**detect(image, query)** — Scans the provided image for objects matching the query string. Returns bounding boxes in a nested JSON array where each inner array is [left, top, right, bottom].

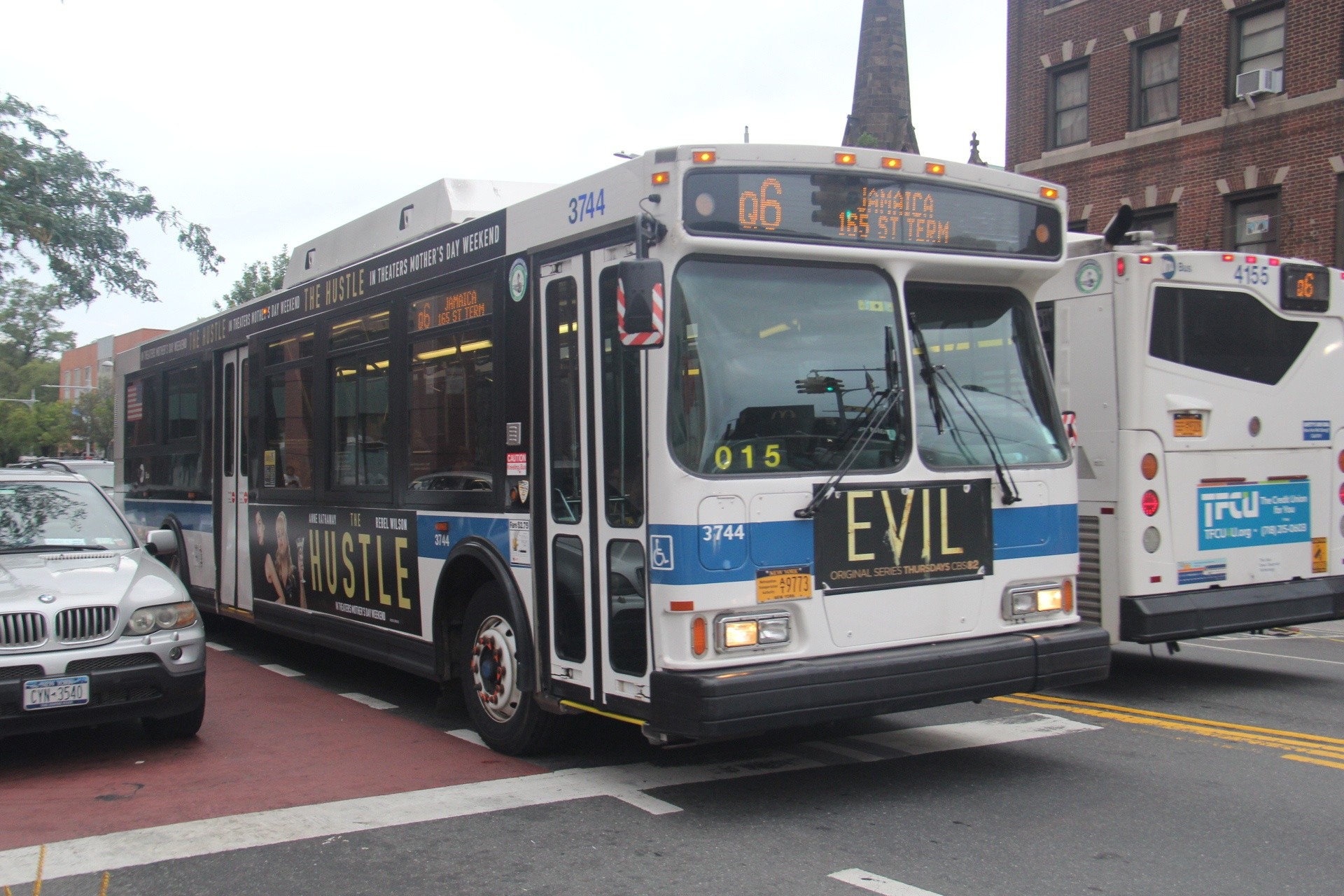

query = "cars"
[[28, 460, 116, 508]]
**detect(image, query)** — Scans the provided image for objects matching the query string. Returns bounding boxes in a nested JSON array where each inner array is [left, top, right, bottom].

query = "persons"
[[250, 509, 308, 609], [283, 466, 303, 488]]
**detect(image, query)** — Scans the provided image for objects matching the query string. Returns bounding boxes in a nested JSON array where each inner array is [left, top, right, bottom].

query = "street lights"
[[41, 366, 99, 460]]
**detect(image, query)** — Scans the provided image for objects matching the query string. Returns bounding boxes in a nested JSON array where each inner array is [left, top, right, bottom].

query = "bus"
[[107, 140, 1114, 763], [912, 203, 1343, 647]]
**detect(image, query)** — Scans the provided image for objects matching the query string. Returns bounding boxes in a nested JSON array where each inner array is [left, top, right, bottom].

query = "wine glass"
[[295, 537, 306, 583]]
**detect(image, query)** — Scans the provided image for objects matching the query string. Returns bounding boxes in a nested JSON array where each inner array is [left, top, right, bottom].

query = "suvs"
[[0, 459, 209, 754]]
